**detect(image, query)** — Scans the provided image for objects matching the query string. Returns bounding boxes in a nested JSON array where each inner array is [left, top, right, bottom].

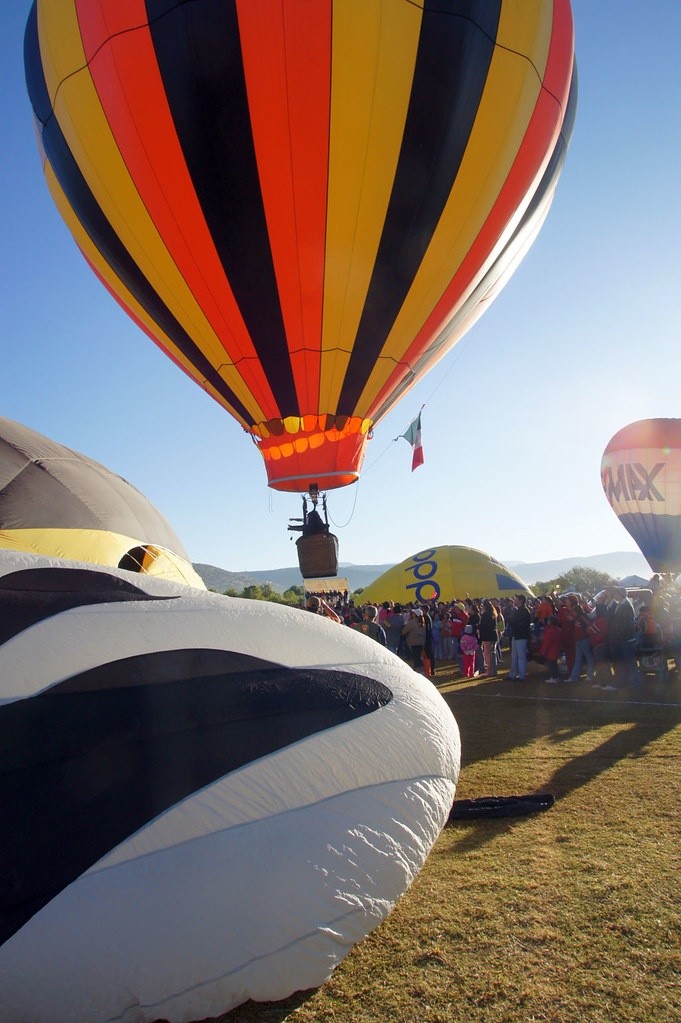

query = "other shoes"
[[563, 678, 575, 682], [602, 686, 618, 691], [592, 684, 602, 688], [584, 677, 592, 681], [503, 676, 515, 680], [513, 678, 523, 682]]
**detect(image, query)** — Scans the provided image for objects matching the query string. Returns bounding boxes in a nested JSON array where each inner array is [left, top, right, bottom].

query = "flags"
[[395, 410, 424, 472]]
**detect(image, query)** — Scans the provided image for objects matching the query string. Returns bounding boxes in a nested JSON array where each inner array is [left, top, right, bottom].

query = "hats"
[[455, 603, 465, 611], [412, 609, 423, 616], [465, 625, 473, 633], [308, 596, 321, 607]]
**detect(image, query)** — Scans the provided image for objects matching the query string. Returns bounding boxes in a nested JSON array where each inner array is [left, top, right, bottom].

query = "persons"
[[305, 575, 681, 691]]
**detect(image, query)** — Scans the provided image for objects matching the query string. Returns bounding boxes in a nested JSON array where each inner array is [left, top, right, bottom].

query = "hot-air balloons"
[[352, 546, 537, 608], [21, 0, 582, 581], [0, 414, 463, 1023], [600, 416, 681, 597]]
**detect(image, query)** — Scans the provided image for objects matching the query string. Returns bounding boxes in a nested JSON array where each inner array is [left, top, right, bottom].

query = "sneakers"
[[545, 677, 560, 683]]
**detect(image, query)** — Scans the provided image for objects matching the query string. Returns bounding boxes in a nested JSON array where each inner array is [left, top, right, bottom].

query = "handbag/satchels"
[[420, 651, 432, 676]]
